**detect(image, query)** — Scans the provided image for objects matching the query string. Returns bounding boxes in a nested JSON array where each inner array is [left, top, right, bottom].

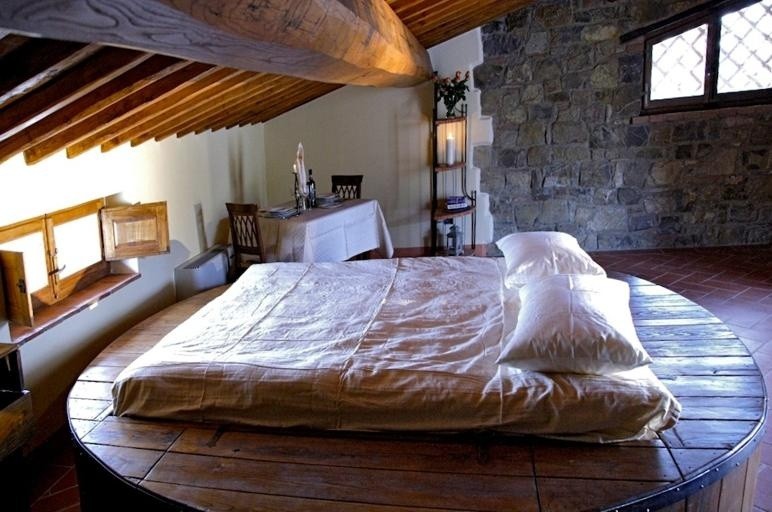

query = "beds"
[[66, 257, 768, 511]]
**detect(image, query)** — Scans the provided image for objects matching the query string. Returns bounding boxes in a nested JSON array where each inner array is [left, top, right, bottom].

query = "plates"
[[316, 193, 337, 199], [320, 203, 343, 208], [267, 207, 292, 213]]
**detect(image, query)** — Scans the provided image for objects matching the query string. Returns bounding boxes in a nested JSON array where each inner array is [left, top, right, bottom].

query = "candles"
[[446, 134, 455, 166]]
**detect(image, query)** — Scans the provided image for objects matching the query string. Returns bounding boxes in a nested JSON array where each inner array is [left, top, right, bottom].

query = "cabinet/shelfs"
[[432, 70, 476, 257]]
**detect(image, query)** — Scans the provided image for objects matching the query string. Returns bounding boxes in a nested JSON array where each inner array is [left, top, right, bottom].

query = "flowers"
[[433, 71, 470, 118]]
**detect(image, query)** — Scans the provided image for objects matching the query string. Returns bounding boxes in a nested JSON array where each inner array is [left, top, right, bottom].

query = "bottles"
[[298, 195, 303, 215], [305, 169, 316, 208]]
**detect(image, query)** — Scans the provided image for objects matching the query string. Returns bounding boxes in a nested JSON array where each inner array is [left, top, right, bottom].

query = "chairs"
[[332, 175, 362, 200], [225, 202, 266, 269]]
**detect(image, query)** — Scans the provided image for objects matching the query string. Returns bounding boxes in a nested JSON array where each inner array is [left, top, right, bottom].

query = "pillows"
[[495, 274, 653, 376], [495, 232, 606, 290]]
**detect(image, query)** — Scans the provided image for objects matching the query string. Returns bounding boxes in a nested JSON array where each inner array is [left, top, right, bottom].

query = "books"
[[258, 206, 298, 219], [317, 192, 345, 209]]
